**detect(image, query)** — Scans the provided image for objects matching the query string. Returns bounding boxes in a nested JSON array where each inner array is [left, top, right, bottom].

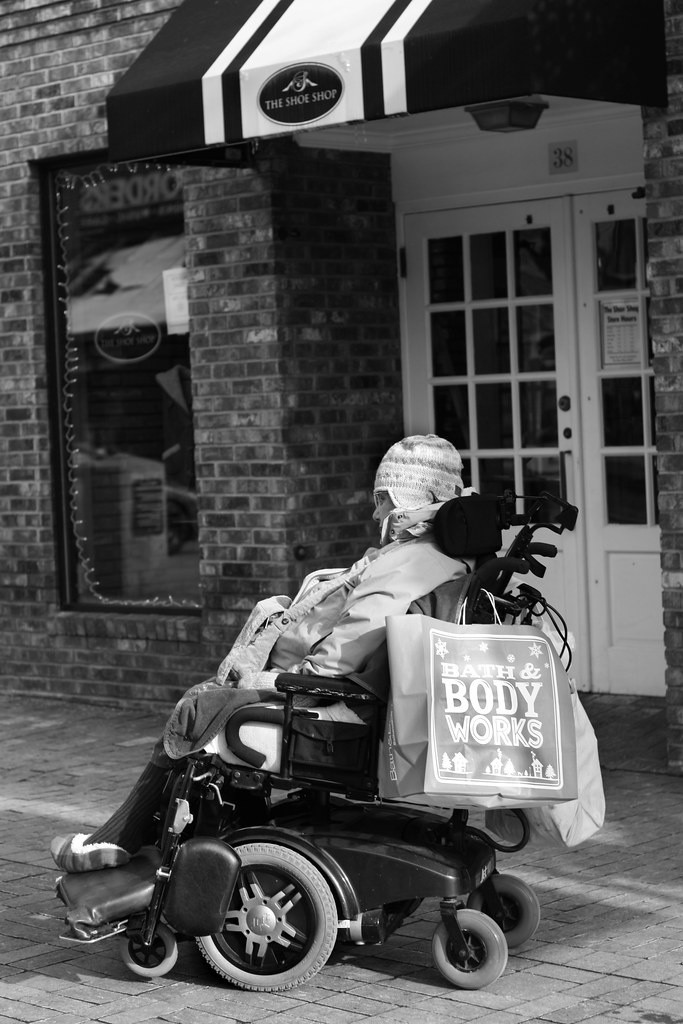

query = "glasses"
[[374, 490, 388, 508]]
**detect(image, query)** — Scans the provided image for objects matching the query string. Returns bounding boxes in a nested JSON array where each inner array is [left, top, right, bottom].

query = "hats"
[[372, 434, 463, 512]]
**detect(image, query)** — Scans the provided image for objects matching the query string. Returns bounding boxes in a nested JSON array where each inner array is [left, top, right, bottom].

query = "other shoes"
[[51, 831, 131, 871]]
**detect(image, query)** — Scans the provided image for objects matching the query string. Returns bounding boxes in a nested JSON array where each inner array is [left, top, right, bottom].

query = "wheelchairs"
[[52, 488, 580, 997]]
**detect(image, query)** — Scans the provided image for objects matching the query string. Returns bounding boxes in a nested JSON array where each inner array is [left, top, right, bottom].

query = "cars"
[[73, 438, 197, 557]]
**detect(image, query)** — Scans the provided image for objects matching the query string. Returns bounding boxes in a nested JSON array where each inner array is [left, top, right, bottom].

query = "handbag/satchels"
[[216, 595, 295, 684], [378, 587, 605, 846]]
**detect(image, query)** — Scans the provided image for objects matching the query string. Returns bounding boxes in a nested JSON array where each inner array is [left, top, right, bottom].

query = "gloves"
[[235, 663, 318, 707]]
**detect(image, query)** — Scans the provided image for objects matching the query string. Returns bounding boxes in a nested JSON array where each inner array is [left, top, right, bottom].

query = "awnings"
[[106, 0, 667, 166]]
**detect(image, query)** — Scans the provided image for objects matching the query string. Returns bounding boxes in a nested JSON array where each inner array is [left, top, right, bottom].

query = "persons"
[[48, 434, 474, 871]]
